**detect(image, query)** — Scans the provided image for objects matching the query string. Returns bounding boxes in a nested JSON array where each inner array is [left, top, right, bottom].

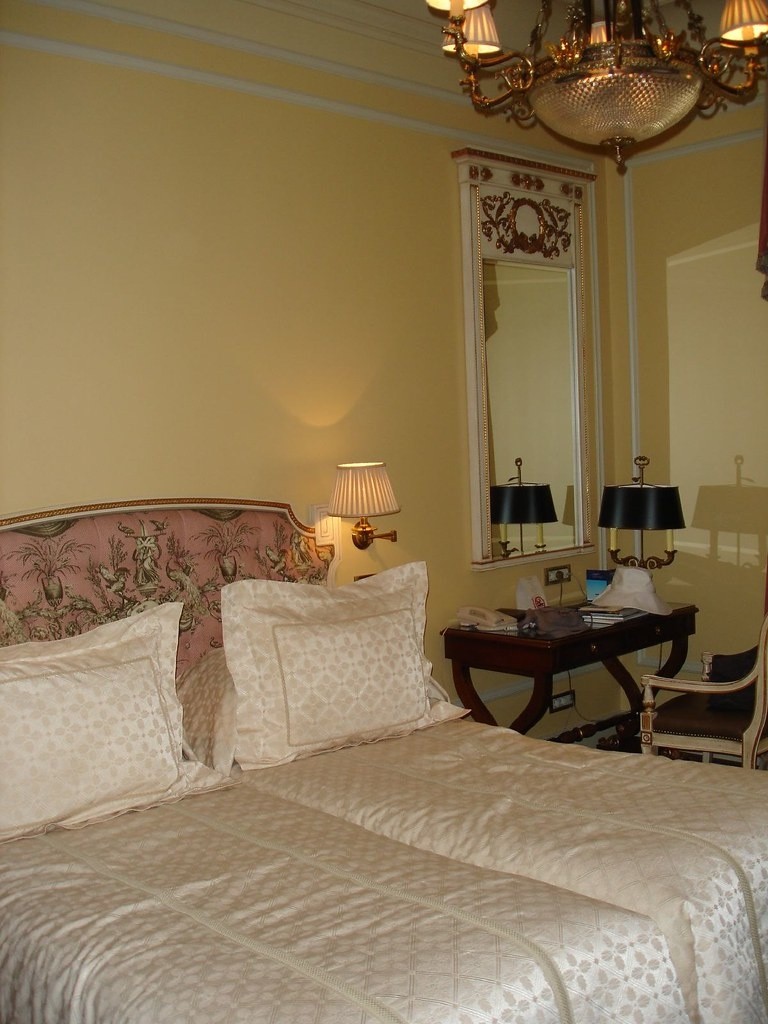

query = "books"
[[580, 609, 648, 620], [584, 619, 621, 625]]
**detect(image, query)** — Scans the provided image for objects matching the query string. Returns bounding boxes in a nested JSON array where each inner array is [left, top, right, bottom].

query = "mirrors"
[[450, 146, 597, 572]]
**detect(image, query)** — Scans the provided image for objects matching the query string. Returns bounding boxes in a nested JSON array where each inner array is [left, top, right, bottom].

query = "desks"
[[439, 597, 699, 759]]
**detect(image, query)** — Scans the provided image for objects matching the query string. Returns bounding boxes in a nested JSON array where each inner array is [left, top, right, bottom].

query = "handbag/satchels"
[[517, 606, 589, 640]]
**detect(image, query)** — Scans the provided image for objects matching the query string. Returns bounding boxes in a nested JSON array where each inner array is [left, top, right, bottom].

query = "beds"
[[0, 497, 768, 1024]]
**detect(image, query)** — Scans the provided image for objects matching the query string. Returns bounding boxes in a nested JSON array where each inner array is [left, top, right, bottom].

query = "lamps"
[[490, 456, 558, 558], [597, 456, 686, 570], [328, 461, 401, 551], [425, 0, 768, 166]]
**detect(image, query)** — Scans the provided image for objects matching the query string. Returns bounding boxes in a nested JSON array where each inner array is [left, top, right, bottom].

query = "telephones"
[[455, 605, 517, 628]]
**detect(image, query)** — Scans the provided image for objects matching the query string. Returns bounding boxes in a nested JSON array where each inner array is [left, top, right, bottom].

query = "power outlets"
[[543, 564, 571, 587], [548, 689, 576, 714]]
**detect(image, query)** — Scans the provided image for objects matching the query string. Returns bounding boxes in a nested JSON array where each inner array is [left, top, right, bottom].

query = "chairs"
[[634, 611, 768, 769]]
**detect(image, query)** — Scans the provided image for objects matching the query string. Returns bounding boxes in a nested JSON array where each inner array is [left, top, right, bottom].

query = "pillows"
[[0, 601, 241, 844], [220, 560, 472, 770]]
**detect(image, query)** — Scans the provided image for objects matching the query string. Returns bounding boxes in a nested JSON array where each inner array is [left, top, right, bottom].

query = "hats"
[[591, 564, 673, 616]]
